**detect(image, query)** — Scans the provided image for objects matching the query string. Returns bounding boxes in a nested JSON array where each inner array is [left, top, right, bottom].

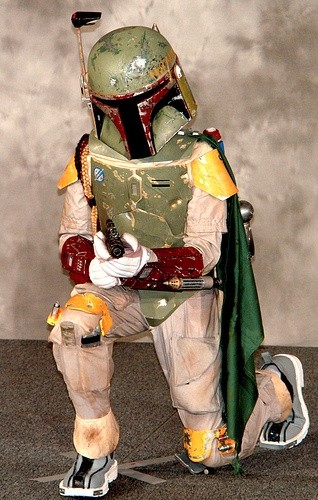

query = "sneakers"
[[58, 449, 118, 498], [259, 352, 309, 450]]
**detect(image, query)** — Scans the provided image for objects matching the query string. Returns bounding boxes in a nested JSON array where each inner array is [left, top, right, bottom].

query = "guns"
[[104, 218, 125, 259]]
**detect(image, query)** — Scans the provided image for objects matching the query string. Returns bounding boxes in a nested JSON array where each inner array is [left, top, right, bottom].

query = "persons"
[[45, 11, 311, 499]]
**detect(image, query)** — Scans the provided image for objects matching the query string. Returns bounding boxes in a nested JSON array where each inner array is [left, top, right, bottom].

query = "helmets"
[[84, 25, 196, 160]]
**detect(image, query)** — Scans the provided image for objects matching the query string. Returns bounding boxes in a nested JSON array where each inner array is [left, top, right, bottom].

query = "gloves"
[[89, 257, 121, 289], [93, 230, 158, 277]]
